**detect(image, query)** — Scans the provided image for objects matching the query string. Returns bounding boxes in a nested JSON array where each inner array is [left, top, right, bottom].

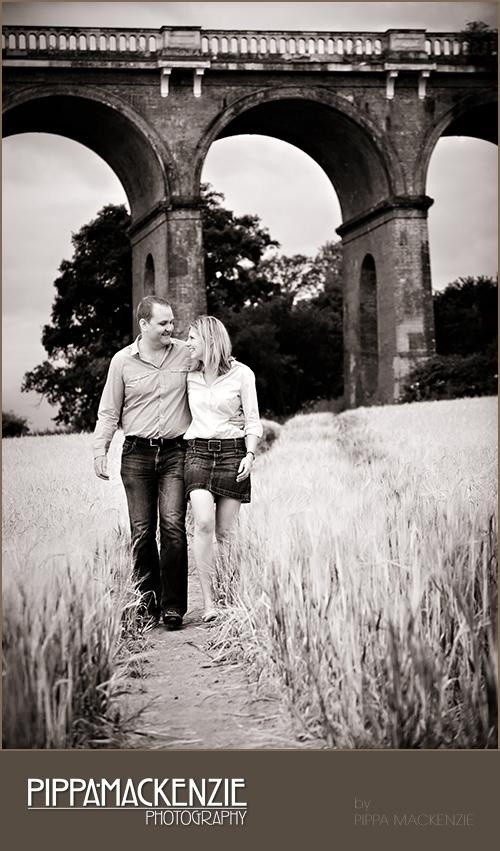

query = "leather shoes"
[[163, 609, 184, 626]]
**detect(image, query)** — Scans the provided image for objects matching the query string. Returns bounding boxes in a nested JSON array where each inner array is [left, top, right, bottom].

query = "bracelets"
[[247, 452, 256, 460]]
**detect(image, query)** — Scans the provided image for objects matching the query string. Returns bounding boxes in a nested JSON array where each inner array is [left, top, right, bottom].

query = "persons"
[[178, 315, 266, 622], [91, 291, 246, 628]]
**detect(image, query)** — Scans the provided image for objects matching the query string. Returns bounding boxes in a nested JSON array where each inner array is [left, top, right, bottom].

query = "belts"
[[186, 439, 243, 451], [125, 436, 182, 447]]
